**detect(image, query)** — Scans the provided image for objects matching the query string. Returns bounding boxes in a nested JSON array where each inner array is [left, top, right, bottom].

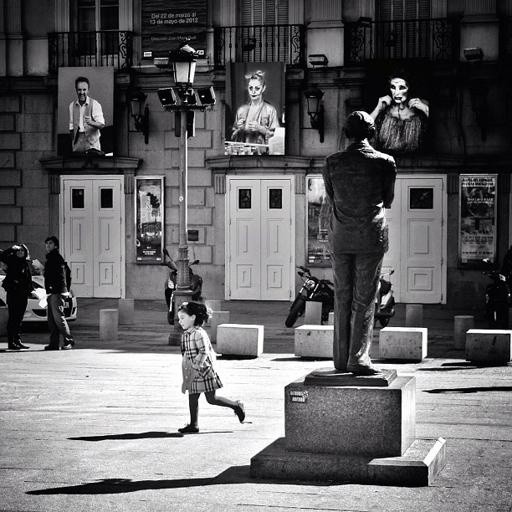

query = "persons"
[[320, 111, 397, 376], [232, 70, 279, 154], [369, 75, 430, 154], [42, 234, 74, 350], [1, 243, 32, 350], [176, 301, 245, 433], [67, 76, 106, 151]]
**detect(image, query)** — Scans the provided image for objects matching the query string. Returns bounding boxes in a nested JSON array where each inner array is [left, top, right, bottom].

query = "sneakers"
[[61, 341, 75, 350], [177, 424, 200, 434], [44, 344, 60, 351], [8, 338, 31, 350], [234, 400, 246, 424]]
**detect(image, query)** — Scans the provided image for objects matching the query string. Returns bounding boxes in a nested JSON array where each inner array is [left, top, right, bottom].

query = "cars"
[[137, 222, 161, 257], [0, 248, 79, 324]]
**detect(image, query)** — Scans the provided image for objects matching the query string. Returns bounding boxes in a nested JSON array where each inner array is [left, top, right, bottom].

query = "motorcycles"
[[159, 250, 202, 324], [283, 265, 396, 327], [481, 257, 512, 328]]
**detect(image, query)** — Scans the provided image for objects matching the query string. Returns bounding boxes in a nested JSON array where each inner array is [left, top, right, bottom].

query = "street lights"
[[159, 38, 217, 345]]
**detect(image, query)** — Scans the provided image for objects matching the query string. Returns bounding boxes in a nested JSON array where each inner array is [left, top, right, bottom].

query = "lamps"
[[126, 90, 152, 145], [304, 82, 327, 144]]
